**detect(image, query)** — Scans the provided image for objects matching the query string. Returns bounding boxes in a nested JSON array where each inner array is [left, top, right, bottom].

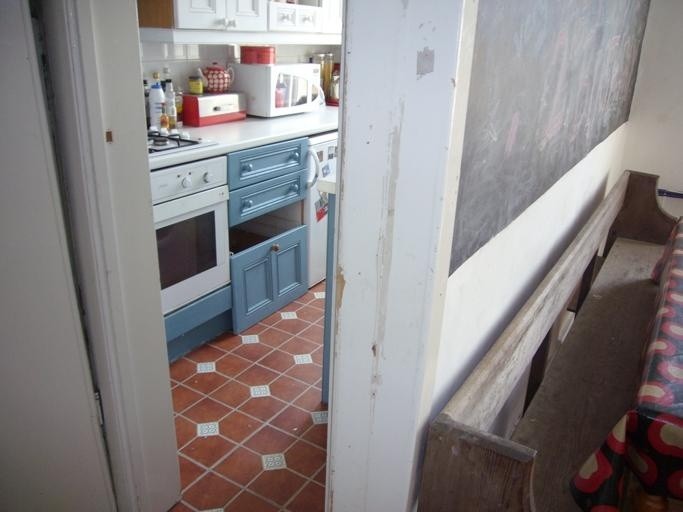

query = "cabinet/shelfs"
[[226, 136, 310, 335], [137, 0, 268, 35], [267, 1, 342, 35]]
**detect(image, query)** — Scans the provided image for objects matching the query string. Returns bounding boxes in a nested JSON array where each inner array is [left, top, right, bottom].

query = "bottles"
[[142, 68, 202, 133]]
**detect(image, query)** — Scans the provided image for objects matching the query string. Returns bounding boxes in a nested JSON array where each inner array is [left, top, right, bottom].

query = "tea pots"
[[195, 61, 235, 95]]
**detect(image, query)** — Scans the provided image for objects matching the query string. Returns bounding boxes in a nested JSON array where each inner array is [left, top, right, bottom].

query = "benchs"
[[417, 167, 676, 511]]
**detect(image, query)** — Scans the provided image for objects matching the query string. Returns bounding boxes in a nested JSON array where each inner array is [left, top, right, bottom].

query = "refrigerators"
[[306, 130, 338, 290]]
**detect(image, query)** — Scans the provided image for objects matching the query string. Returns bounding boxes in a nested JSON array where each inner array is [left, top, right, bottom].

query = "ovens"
[[149, 156, 231, 316]]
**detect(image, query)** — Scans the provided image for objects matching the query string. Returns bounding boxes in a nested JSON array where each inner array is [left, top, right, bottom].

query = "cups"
[[311, 52, 334, 102]]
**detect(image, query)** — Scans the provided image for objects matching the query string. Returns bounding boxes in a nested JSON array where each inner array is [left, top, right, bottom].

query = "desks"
[[632, 217, 681, 500]]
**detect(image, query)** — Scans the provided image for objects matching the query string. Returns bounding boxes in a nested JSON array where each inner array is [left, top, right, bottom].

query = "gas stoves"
[[146, 126, 219, 157]]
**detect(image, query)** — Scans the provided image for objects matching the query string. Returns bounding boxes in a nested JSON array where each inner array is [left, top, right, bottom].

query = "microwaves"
[[225, 63, 321, 118]]
